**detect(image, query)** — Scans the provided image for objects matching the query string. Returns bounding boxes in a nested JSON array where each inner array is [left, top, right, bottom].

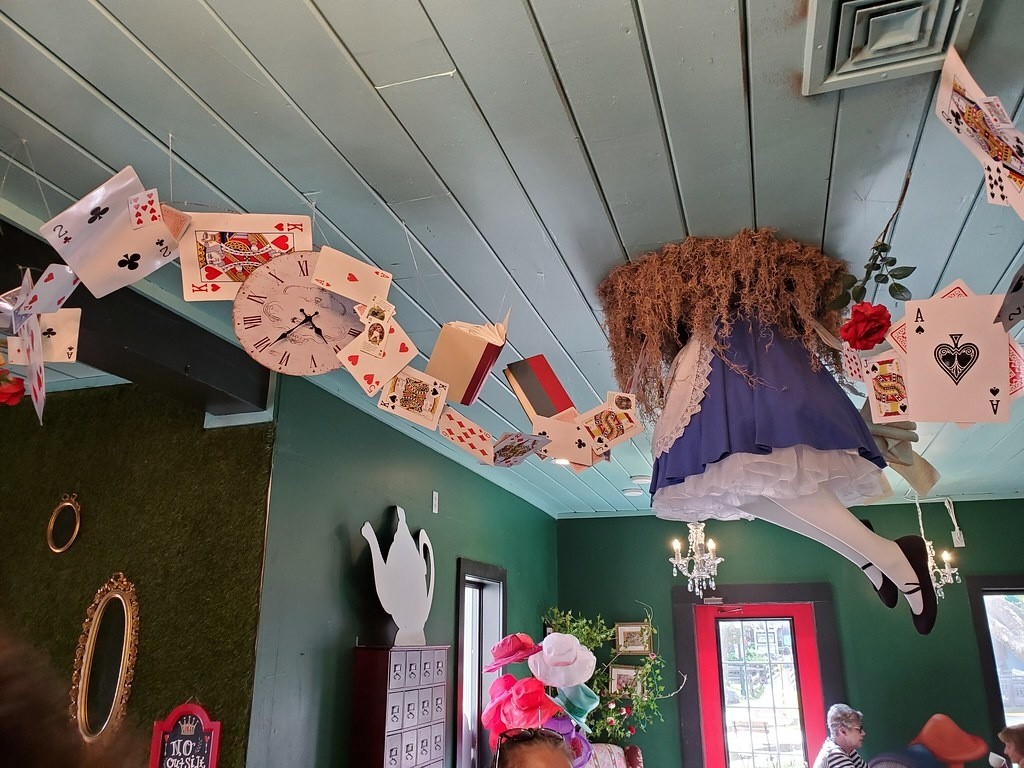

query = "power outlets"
[[950, 530, 966, 548]]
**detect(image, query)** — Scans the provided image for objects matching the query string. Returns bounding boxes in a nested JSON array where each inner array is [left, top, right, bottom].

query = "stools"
[[907, 713, 989, 767]]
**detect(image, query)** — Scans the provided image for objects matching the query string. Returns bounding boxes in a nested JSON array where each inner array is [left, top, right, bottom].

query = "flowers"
[[822, 174, 917, 350], [0, 367, 26, 408], [593, 653, 663, 741]]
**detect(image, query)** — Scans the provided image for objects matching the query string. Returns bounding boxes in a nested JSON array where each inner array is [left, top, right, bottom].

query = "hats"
[[545, 683, 600, 734], [481, 674, 561, 752], [480, 633, 544, 673], [543, 717, 592, 768], [527, 632, 596, 687]]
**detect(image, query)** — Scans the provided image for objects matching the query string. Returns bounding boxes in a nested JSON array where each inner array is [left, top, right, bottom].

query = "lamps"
[[913, 493, 962, 604], [667, 522, 725, 600]]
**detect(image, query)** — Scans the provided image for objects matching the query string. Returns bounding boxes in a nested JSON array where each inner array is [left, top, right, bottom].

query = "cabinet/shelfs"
[[348, 644, 452, 767]]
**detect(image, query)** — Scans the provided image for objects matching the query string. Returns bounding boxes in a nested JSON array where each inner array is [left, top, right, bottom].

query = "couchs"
[[582, 744, 644, 768]]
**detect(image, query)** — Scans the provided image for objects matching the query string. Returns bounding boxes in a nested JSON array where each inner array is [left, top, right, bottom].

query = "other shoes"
[[858, 519, 897, 609], [893, 535, 937, 636]]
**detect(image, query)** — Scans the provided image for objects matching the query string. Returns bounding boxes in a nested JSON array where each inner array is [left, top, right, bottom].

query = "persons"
[[494, 727, 572, 768], [813, 703, 870, 768], [998, 723, 1024, 768], [649, 324, 937, 635]]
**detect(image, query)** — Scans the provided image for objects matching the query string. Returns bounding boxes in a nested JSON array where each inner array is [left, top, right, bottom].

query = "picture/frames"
[[609, 665, 648, 699], [615, 623, 653, 656]]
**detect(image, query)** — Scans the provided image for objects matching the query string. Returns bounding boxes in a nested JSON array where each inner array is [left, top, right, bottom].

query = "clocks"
[[232, 251, 370, 376]]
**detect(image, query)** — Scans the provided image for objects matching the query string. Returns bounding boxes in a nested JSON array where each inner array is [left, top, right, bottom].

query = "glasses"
[[846, 724, 862, 733], [496, 727, 564, 768]]
